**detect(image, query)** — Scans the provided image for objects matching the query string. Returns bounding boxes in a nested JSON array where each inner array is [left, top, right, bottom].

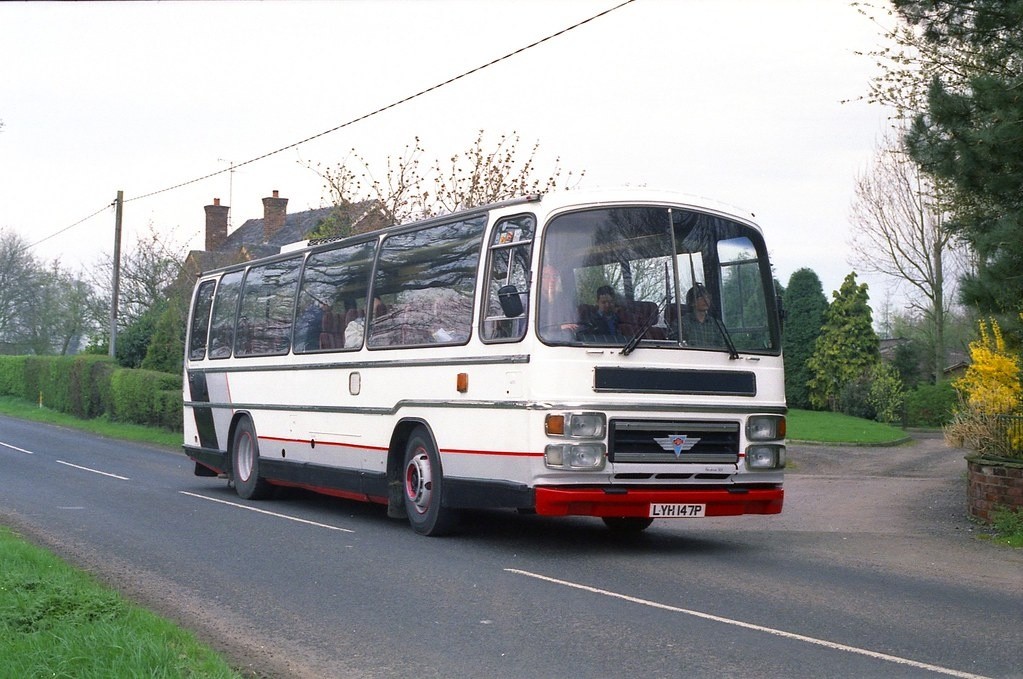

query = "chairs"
[[218, 301, 688, 358]]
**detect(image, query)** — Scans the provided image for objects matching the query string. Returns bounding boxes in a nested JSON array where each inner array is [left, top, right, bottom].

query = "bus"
[[181, 188, 788, 538]]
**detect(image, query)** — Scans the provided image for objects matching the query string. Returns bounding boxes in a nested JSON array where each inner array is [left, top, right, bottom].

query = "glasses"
[[696, 294, 712, 300]]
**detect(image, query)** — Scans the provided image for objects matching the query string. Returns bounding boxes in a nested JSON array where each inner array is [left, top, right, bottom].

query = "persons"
[[344, 293, 382, 349], [538, 265, 581, 343], [670, 285, 732, 350], [587, 285, 630, 337]]
